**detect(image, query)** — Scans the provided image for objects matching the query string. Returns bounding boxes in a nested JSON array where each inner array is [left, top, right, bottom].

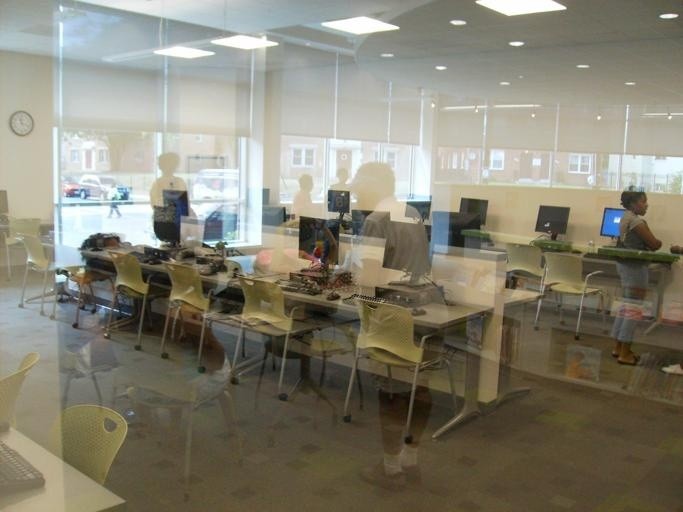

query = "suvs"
[[80, 176, 130, 199]]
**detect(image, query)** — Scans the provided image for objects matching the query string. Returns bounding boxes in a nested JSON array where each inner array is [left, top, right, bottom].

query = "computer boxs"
[[375, 283, 443, 307], [290, 268, 351, 289], [145, 245, 186, 260], [196, 254, 225, 265]]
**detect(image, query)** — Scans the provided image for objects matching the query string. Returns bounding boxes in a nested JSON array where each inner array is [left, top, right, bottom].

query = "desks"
[[79, 243, 546, 442], [0, 430, 125, 511]]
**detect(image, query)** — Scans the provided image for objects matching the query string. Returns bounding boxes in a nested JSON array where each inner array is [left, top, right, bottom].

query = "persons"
[[612, 185, 662, 364], [662, 246, 683, 375], [294, 174, 321, 221], [481, 168, 489, 184], [354, 162, 447, 485], [329, 166, 352, 192], [150, 152, 198, 241], [108, 186, 121, 217]]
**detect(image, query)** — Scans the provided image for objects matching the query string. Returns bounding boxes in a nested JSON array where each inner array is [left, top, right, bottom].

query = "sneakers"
[[660, 364, 682, 375], [360, 464, 407, 491], [403, 464, 422, 486]]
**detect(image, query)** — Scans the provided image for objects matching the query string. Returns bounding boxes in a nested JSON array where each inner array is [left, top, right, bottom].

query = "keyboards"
[[343, 294, 411, 311], [128, 251, 155, 263], [274, 279, 323, 295]]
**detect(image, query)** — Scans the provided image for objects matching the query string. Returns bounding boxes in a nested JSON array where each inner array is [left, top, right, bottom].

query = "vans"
[[192, 169, 240, 202]]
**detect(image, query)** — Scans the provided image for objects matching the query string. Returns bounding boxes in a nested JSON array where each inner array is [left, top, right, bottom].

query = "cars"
[[63, 182, 101, 198]]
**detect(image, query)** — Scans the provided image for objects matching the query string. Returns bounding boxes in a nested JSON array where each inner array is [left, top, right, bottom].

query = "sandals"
[[617, 355, 643, 366]]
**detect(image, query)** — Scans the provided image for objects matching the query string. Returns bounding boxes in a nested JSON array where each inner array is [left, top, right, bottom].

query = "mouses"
[[411, 308, 426, 316], [327, 292, 340, 300], [199, 265, 218, 275], [148, 260, 161, 265]]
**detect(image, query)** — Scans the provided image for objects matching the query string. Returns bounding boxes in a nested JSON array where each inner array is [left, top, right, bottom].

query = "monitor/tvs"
[[460, 197, 489, 225], [328, 190, 349, 212], [299, 216, 342, 265], [352, 210, 390, 238], [405, 201, 431, 217], [600, 208, 628, 238], [432, 211, 481, 249], [162, 189, 187, 216], [153, 206, 180, 242], [0, 190, 9, 213], [383, 221, 431, 281], [535, 205, 570, 234], [262, 206, 286, 225]]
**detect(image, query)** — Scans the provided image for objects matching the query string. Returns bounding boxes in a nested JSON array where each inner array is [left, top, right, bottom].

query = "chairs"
[[159, 261, 246, 376], [42, 404, 127, 487], [342, 298, 458, 445], [1, 351, 39, 433], [230, 277, 331, 403], [533, 249, 608, 341], [507, 267, 561, 330], [8, 231, 72, 318], [103, 250, 172, 349]]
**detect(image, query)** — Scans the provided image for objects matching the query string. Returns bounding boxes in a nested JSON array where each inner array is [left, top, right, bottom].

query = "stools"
[[53, 266, 114, 332]]
